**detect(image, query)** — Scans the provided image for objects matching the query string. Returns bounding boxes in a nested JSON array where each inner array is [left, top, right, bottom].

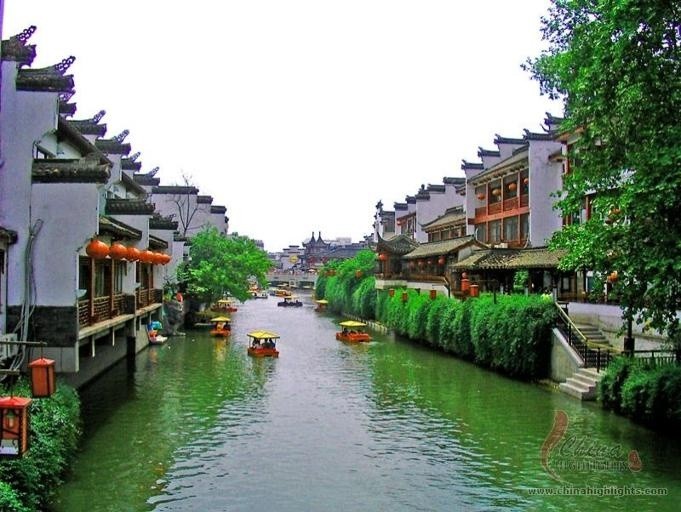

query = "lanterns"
[[86, 239, 171, 266], [409, 258, 445, 268], [607, 272, 617, 284], [476, 192, 485, 201], [380, 255, 384, 259], [492, 188, 500, 196], [461, 272, 468, 278], [523, 178, 528, 184], [509, 183, 517, 191], [608, 208, 619, 220]]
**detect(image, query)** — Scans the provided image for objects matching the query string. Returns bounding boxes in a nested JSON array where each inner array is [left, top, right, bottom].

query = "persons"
[[549, 290, 554, 298], [254, 293, 257, 296], [223, 322, 230, 331], [540, 287, 549, 299], [252, 337, 275, 347], [343, 328, 349, 336], [177, 291, 183, 309], [358, 329, 365, 334]]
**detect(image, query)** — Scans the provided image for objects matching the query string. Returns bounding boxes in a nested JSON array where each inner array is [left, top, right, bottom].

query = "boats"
[[246, 331, 281, 358], [315, 300, 328, 312], [336, 321, 371, 341], [247, 286, 303, 306], [211, 299, 238, 312], [210, 317, 231, 336]]
